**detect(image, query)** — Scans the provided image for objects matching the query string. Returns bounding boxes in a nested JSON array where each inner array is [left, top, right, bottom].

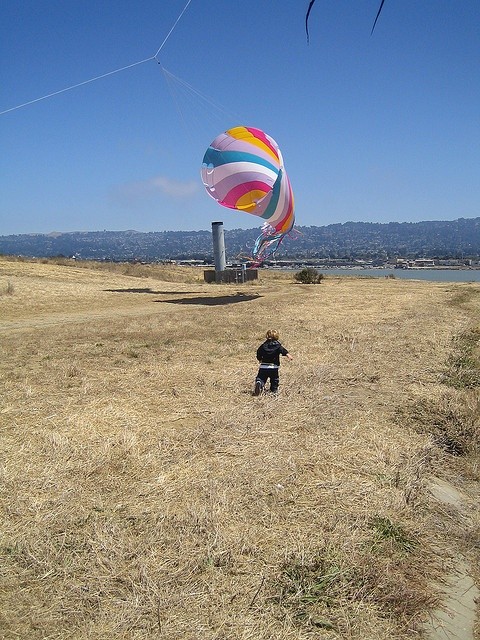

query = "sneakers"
[[255, 381, 261, 396]]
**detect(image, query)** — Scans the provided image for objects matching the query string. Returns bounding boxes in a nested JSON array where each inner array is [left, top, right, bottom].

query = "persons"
[[255, 329, 293, 395]]
